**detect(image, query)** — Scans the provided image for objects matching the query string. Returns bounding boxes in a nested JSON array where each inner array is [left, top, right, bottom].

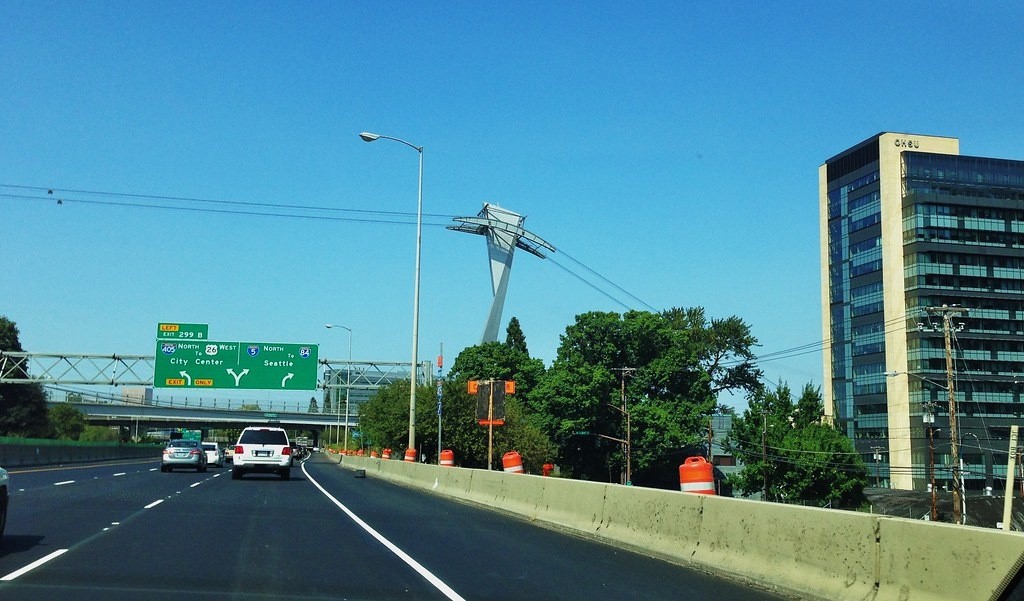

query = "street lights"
[[359, 130, 424, 460], [326, 322, 353, 452]]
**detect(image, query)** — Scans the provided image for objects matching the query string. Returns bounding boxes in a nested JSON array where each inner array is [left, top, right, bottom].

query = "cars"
[[161, 440, 207, 472], [200, 441, 224, 467], [219, 445, 236, 461]]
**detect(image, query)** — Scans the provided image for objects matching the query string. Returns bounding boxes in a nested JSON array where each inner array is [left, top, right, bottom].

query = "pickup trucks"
[[231, 427, 293, 480]]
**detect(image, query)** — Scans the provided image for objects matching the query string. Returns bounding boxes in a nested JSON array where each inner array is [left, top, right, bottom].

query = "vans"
[[289, 441, 318, 459]]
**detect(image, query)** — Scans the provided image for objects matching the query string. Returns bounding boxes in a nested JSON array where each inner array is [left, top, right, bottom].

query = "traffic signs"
[[154, 338, 319, 389]]
